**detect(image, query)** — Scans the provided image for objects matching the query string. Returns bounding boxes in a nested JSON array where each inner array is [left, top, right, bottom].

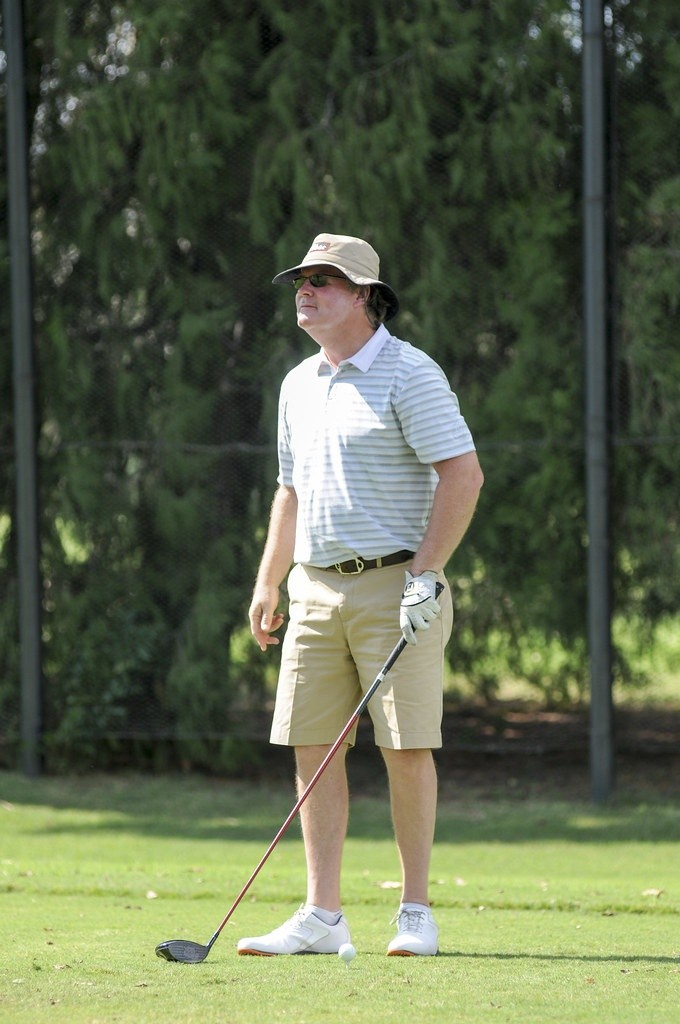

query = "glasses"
[[292, 273, 348, 289]]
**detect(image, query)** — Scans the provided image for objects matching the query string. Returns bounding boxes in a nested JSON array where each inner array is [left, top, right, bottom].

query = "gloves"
[[399, 569, 441, 646]]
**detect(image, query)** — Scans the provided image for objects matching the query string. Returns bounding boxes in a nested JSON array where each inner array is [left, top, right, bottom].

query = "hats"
[[272, 232, 399, 324]]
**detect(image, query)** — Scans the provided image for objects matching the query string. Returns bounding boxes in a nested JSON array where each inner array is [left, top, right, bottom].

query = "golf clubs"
[[154, 580, 445, 965]]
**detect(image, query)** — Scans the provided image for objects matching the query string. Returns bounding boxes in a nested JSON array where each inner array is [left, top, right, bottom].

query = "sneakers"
[[387, 906, 439, 957], [236, 903, 351, 956]]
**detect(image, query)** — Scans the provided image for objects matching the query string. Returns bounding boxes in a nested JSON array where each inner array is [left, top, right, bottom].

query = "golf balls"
[[339, 943, 357, 962]]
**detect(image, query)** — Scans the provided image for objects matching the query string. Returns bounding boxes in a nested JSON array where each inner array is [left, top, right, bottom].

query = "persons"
[[238, 235, 484, 953]]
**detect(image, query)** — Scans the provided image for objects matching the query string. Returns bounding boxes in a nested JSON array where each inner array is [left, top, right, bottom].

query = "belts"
[[328, 549, 415, 574]]
